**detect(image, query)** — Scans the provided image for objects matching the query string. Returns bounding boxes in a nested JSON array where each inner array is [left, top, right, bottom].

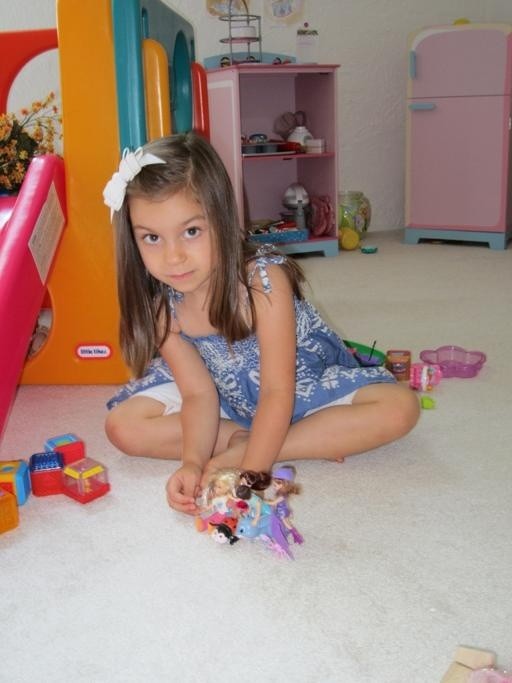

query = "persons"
[[191, 463, 304, 560], [102, 131, 420, 485]]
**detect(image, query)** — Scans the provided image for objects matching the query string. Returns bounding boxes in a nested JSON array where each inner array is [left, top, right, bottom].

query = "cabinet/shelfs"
[[205, 63, 339, 264], [402, 22, 510, 250]]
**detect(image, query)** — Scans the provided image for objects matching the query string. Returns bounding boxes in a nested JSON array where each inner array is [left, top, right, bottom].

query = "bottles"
[[295, 199, 306, 229], [287, 125, 314, 153]]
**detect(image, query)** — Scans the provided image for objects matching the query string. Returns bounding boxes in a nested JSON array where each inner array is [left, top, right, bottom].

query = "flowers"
[[0, 91, 63, 196]]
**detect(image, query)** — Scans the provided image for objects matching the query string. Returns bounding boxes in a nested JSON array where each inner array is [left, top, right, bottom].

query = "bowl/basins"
[[420, 344, 485, 378]]
